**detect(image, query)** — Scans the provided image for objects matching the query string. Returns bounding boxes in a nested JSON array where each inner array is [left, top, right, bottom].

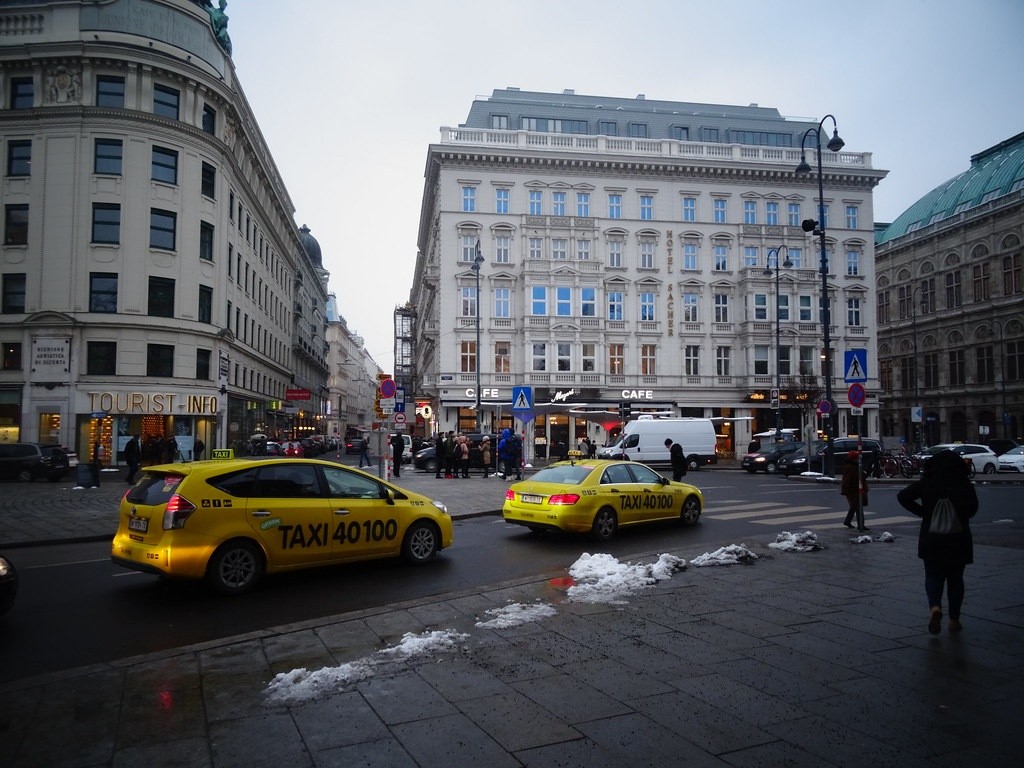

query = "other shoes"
[[949, 618, 961, 631], [435, 472, 521, 481], [929, 605, 943, 634], [858, 524, 870, 531], [844, 522, 854, 528]]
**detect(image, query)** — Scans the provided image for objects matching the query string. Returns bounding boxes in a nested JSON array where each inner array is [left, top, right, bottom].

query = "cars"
[[345, 438, 368, 455], [0, 557, 19, 617], [741, 441, 807, 474], [997, 445, 1024, 474], [911, 443, 1000, 475], [266, 434, 343, 458], [111, 449, 454, 596], [503, 450, 705, 544]]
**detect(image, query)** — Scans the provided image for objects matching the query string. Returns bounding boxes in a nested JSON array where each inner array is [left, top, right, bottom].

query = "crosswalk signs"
[[844, 349, 868, 383], [513, 387, 532, 411]]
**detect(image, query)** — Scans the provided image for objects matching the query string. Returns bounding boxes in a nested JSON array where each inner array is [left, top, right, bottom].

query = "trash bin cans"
[[76, 463, 99, 488]]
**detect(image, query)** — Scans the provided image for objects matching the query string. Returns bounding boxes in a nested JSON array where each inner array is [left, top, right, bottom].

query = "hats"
[[665, 439, 672, 444], [848, 450, 858, 459], [483, 436, 489, 442]]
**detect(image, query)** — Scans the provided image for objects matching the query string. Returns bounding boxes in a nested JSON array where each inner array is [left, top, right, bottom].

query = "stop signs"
[[395, 412, 406, 423]]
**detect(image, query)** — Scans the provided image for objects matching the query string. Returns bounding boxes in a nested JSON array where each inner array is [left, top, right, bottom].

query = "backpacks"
[[505, 439, 515, 456], [455, 443, 463, 458], [928, 497, 962, 534]]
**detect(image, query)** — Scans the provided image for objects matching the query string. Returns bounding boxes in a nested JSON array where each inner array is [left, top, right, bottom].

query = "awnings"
[[351, 426, 370, 433]]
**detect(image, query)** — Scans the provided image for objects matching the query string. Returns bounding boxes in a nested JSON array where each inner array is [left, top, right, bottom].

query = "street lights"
[[988, 320, 1008, 440], [470, 239, 485, 434], [795, 114, 846, 436], [762, 244, 794, 442]]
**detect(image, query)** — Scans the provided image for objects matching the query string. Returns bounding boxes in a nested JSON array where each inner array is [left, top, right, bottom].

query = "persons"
[[359, 437, 372, 467], [840, 450, 870, 530], [498, 428, 521, 480], [124, 434, 141, 484], [259, 436, 267, 456], [411, 435, 436, 460], [478, 435, 491, 478], [664, 438, 688, 482], [897, 449, 979, 635], [435, 430, 472, 479], [193, 436, 204, 461], [748, 438, 760, 454], [142, 434, 177, 466], [580, 437, 597, 459], [391, 430, 404, 477]]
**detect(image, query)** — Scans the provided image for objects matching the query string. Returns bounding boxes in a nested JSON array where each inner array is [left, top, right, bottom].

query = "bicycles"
[[956, 451, 976, 479], [873, 447, 924, 478]]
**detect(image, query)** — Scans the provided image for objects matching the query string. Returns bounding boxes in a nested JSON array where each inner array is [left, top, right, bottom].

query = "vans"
[[414, 434, 523, 473], [597, 414, 719, 471], [389, 433, 412, 464], [776, 436, 885, 477]]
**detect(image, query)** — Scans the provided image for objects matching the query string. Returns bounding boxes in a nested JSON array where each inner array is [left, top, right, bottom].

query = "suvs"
[[0, 442, 71, 484], [60, 446, 80, 477]]
